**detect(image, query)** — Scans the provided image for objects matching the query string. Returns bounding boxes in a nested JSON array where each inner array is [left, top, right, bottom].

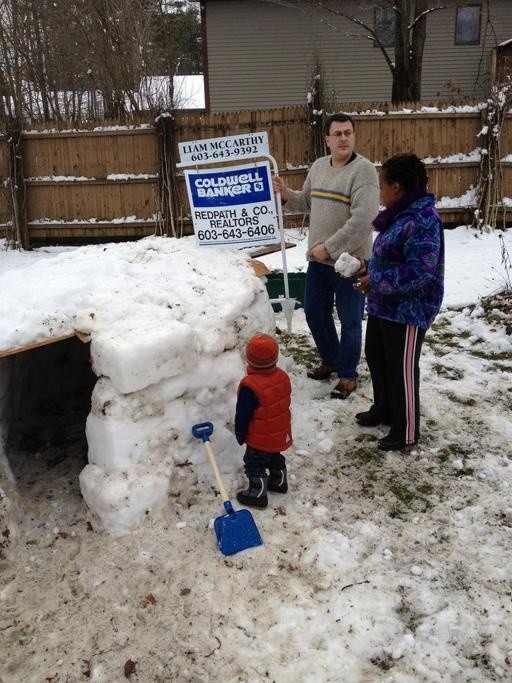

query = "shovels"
[[193, 422, 262, 557]]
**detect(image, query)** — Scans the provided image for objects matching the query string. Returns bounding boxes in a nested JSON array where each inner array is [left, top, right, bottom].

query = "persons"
[[233, 332, 293, 509], [272, 112, 381, 399], [338, 151, 445, 450]]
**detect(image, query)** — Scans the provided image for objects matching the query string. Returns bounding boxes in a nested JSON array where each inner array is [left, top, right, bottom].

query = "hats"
[[244, 330, 279, 368]]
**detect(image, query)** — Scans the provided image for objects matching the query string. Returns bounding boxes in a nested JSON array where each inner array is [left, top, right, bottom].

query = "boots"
[[237, 473, 268, 510], [268, 466, 288, 494]]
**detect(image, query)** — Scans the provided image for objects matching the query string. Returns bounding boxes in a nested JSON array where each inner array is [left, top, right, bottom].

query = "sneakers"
[[354, 404, 383, 426], [306, 362, 337, 381], [330, 378, 357, 400], [377, 435, 403, 451]]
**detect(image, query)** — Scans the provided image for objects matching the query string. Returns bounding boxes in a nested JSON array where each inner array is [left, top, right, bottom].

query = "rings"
[[355, 282, 361, 286]]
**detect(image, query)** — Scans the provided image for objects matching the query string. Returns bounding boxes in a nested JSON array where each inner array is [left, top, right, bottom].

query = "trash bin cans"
[[265, 272, 307, 312]]
